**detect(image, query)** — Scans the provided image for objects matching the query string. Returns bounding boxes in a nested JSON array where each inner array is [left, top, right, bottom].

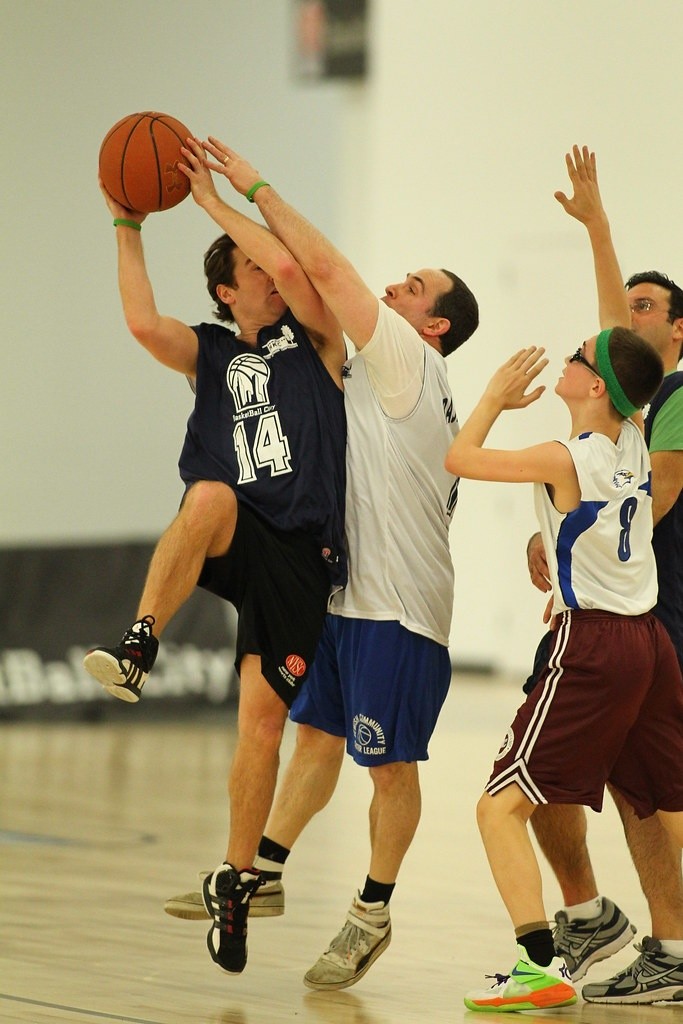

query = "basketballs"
[[99, 110, 195, 212]]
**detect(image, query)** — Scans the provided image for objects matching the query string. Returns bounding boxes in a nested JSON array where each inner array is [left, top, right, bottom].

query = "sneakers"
[[163, 881, 286, 921], [582, 936, 683, 1004], [463, 944, 578, 1011], [83, 614, 159, 703], [302, 890, 393, 991], [548, 897, 637, 983], [202, 862, 267, 976]]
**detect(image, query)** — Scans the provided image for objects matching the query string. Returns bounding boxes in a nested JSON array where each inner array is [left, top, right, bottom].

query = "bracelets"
[[246, 181, 270, 203], [113, 219, 141, 231]]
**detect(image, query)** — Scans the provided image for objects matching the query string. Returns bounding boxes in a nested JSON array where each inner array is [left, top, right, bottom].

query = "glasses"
[[630, 301, 678, 316], [570, 348, 606, 392]]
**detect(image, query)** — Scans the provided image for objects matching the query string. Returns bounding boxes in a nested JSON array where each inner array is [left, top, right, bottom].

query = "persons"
[[164, 135, 479, 991], [82, 138, 348, 976], [526, 270, 683, 1004], [444, 145, 683, 1013]]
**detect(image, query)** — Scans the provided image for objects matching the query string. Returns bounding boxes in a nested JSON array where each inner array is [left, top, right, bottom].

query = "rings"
[[223, 156, 229, 164]]
